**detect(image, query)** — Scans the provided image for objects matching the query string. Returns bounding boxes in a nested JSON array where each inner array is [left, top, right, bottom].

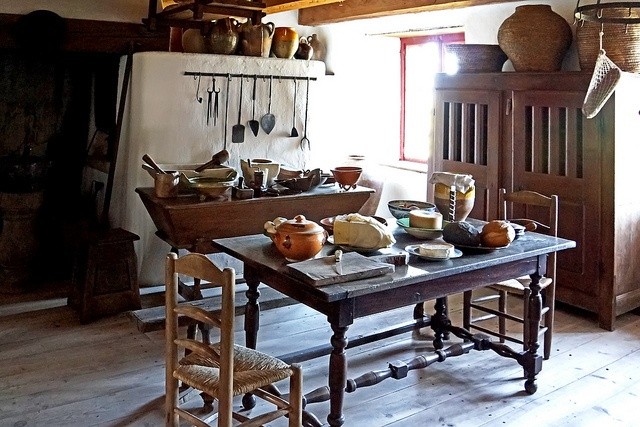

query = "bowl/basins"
[[181, 170, 238, 201], [320, 215, 387, 236], [396, 217, 451, 239], [330, 167, 363, 186], [388, 198, 437, 218]]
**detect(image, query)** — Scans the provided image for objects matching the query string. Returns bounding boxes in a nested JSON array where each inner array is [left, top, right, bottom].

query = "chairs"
[[461, 183, 560, 361], [160, 251, 302, 427]]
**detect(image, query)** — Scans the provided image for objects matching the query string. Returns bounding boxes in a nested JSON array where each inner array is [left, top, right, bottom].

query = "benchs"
[[124, 275, 428, 390]]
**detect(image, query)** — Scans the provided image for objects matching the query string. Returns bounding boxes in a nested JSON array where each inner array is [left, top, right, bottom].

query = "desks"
[[132, 172, 376, 302]]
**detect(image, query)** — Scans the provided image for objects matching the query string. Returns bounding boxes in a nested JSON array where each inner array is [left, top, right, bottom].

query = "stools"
[[66, 227, 143, 322]]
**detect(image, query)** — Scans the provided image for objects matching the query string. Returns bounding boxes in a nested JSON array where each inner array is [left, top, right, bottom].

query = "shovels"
[[288, 78, 301, 137], [261, 76, 275, 135], [249, 76, 259, 137], [232, 76, 245, 143]]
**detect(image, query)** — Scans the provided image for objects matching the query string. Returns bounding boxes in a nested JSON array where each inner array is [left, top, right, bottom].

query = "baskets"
[[447, 43, 508, 73]]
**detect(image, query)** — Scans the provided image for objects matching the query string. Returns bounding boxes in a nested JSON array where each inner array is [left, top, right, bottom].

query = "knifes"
[[335, 249, 343, 276]]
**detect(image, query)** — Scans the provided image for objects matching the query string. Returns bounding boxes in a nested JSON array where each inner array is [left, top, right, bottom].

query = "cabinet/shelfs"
[[417, 68, 639, 333]]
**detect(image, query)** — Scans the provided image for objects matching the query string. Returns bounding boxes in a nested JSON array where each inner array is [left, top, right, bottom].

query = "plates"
[[442, 237, 510, 252], [327, 234, 384, 253], [405, 243, 463, 261]]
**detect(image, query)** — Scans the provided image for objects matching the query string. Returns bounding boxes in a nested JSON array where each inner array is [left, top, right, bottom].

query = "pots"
[[264, 214, 329, 263]]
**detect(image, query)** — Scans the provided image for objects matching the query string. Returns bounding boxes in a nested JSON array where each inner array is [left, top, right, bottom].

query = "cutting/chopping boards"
[[285, 250, 407, 287]]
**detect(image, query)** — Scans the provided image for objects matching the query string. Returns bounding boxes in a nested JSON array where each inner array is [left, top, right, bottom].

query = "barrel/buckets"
[[497, 5, 573, 72]]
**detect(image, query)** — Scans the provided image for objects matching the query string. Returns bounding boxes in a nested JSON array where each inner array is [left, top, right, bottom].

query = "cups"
[[155, 170, 179, 198]]
